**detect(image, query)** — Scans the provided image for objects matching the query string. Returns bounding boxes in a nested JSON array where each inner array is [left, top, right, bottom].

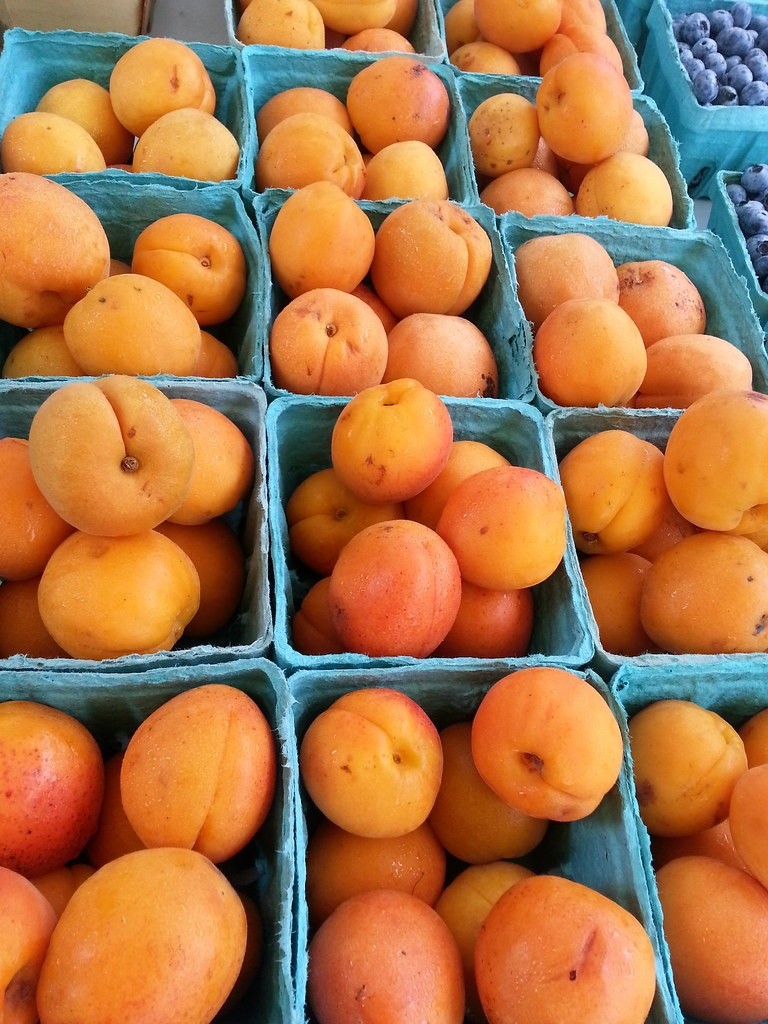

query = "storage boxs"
[[0, 0, 767, 1024]]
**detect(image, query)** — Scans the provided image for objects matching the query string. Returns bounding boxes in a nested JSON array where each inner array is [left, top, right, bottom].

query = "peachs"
[[0, 0, 767, 1024]]
